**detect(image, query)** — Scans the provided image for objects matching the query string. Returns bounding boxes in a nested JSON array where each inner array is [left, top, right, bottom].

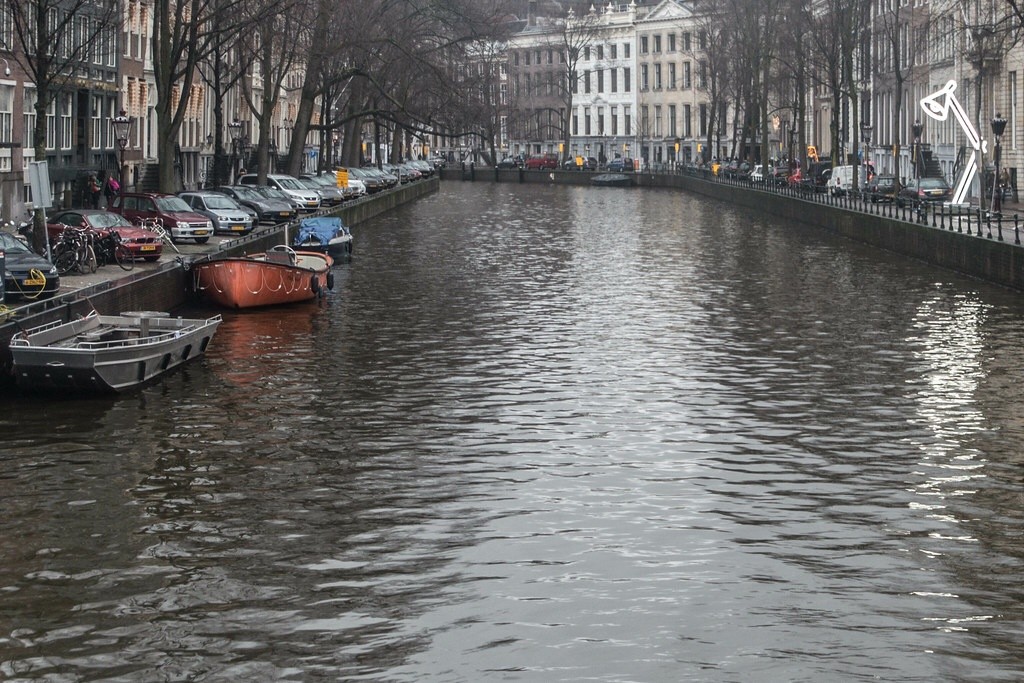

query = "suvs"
[[807, 161, 832, 192]]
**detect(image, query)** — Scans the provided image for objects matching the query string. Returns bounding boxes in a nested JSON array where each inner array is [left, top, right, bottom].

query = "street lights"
[[862, 123, 873, 201], [913, 120, 923, 205], [332, 129, 338, 168], [111, 113, 134, 215], [990, 113, 1007, 214], [228, 116, 242, 184]]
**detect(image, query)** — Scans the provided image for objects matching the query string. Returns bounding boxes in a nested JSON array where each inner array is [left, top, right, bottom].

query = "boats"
[[187, 245, 335, 305], [295, 218, 354, 257], [9, 307, 224, 392]]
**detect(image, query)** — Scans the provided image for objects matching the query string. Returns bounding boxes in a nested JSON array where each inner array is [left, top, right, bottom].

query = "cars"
[[498, 153, 598, 171], [862, 174, 901, 203], [181, 184, 295, 235], [1, 230, 60, 298], [298, 157, 447, 208], [238, 172, 320, 213], [896, 176, 951, 207], [46, 210, 163, 263], [108, 191, 214, 242], [607, 157, 634, 173], [706, 165, 802, 190]]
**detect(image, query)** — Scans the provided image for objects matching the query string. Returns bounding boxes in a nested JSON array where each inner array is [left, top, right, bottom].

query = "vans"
[[827, 165, 862, 198]]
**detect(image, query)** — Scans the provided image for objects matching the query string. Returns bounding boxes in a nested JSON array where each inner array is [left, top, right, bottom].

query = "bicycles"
[[0, 213, 135, 272], [136, 218, 180, 254]]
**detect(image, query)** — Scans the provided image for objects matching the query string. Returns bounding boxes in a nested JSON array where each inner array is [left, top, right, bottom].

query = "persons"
[[108, 175, 119, 195], [601, 155, 607, 166], [867, 166, 876, 181], [89, 174, 102, 210], [197, 169, 205, 190], [235, 168, 245, 186], [1000, 167, 1009, 187], [568, 154, 572, 160]]
